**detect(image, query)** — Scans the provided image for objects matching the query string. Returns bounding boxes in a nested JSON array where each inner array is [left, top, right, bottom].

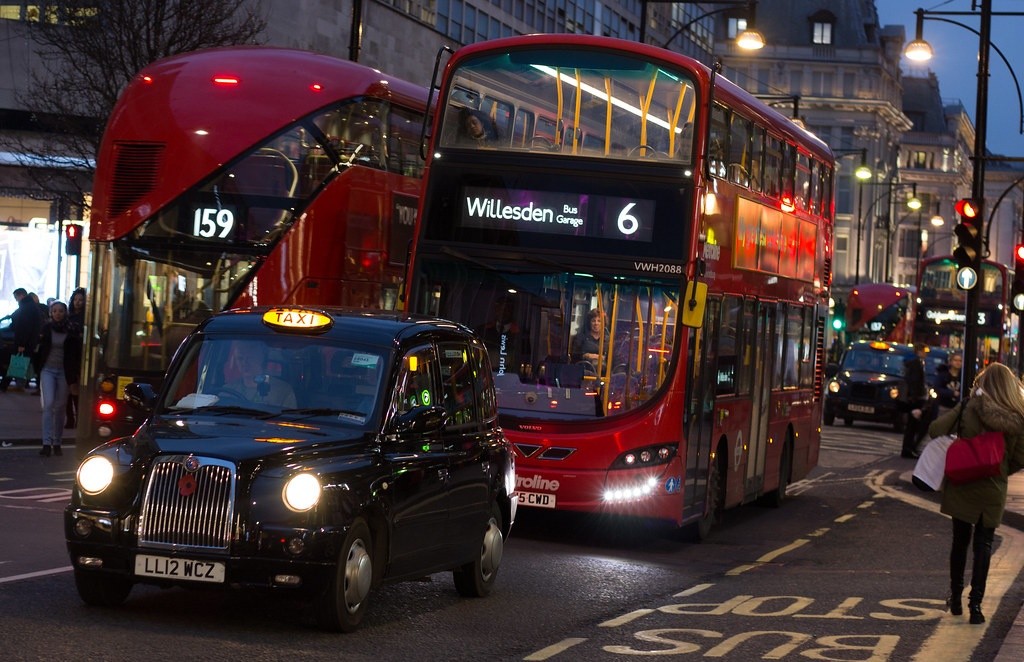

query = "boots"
[[64, 393, 78, 428]]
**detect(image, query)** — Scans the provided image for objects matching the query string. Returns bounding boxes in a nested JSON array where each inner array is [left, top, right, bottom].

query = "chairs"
[[628, 144, 670, 158], [234, 137, 372, 240], [523, 135, 553, 150], [576, 359, 638, 392], [331, 351, 368, 376]]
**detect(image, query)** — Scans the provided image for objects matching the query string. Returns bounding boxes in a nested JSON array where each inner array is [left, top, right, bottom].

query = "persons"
[[472, 295, 530, 377], [31, 299, 83, 455], [216, 338, 297, 408], [465, 113, 490, 144], [675, 122, 695, 163], [0, 286, 87, 428], [902, 343, 929, 459], [570, 308, 612, 369], [931, 351, 963, 409], [928, 362, 1024, 625]]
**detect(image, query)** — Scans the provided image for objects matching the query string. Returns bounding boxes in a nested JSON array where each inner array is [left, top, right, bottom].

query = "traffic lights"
[[1012, 244, 1024, 291], [833, 303, 845, 330], [65, 224, 82, 256], [953, 197, 986, 272]]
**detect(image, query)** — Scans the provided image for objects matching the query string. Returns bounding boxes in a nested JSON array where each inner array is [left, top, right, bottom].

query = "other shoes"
[[39, 445, 52, 456], [946, 593, 963, 615], [53, 445, 62, 455], [967, 602, 986, 624], [901, 449, 922, 457]]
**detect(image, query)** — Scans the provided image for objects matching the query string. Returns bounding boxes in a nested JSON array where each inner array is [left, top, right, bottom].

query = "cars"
[[825, 340, 959, 434], [64, 304, 519, 635]]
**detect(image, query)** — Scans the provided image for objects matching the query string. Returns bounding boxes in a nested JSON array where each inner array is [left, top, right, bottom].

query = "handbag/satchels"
[[6, 351, 30, 379], [943, 397, 1007, 482], [912, 397, 970, 493]]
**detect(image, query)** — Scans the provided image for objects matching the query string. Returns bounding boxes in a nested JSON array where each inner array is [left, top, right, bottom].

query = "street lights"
[[902, 0, 1024, 405], [637, 1, 766, 53], [854, 181, 921, 289], [885, 200, 945, 285]]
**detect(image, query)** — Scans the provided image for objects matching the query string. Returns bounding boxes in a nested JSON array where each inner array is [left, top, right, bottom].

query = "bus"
[[844, 282, 918, 350], [401, 31, 836, 543], [75, 44, 569, 465], [915, 255, 1015, 387]]
